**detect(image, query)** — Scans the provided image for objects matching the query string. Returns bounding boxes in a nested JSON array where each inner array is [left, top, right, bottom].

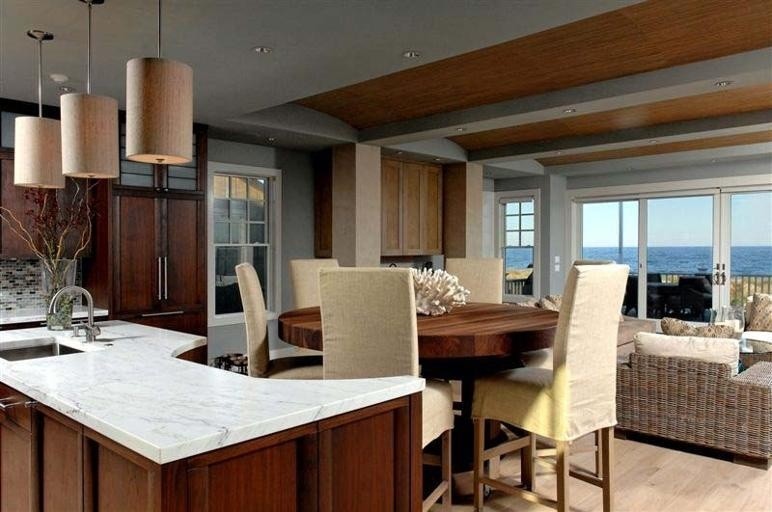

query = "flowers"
[[0, 172, 102, 326]]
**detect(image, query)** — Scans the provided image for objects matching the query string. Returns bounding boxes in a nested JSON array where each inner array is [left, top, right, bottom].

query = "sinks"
[[0, 337, 84, 361]]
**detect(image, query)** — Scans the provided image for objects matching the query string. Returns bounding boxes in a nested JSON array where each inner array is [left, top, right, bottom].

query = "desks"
[[278, 297, 561, 505]]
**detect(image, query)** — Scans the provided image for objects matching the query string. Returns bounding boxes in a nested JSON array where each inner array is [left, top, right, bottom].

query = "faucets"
[[48, 285, 101, 342]]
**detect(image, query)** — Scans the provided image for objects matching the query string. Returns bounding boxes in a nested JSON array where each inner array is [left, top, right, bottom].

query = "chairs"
[[492, 257, 617, 370], [290, 258, 344, 311], [470, 265, 631, 512], [445, 257, 505, 303], [317, 265, 455, 512], [236, 263, 323, 379]]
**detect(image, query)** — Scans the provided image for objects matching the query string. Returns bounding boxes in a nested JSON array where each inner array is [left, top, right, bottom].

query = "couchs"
[[619, 268, 716, 323], [614, 341, 771, 463]]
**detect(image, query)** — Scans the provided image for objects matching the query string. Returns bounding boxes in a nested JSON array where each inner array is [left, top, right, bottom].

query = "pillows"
[[660, 316, 741, 344], [746, 290, 772, 334], [634, 331, 739, 376]]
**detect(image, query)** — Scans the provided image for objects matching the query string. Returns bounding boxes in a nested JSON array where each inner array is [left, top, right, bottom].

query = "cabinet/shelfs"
[[1, 146, 96, 260], [28, 403, 161, 511], [0, 381, 31, 512], [107, 109, 208, 195], [382, 157, 443, 255], [81, 191, 208, 367]]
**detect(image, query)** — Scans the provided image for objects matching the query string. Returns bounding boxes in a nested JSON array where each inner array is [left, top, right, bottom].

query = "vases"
[[39, 257, 79, 331]]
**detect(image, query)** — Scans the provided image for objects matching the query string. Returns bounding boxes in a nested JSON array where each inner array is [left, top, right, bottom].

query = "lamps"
[[11, 29, 66, 189], [124, 1, 192, 166], [59, 0, 121, 179]]
